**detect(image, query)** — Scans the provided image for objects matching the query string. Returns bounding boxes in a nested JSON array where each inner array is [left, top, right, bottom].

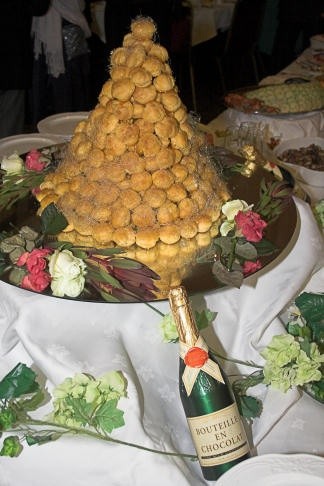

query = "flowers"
[[1, 145, 324, 458]]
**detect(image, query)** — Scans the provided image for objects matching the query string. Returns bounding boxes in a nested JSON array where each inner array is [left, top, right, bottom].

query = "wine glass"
[[189, 111, 201, 128]]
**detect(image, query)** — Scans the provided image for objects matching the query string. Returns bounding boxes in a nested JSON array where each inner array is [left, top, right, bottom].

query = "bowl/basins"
[[38, 111, 91, 159], [273, 136, 324, 201], [0, 134, 65, 175]]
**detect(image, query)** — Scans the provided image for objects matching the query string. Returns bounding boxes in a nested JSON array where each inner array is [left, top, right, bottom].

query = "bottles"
[[242, 145, 311, 205], [168, 286, 252, 481], [240, 160, 257, 178]]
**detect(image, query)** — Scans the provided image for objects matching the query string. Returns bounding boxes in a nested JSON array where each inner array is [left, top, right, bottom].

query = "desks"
[[208, 49, 324, 161], [89, 0, 235, 117]]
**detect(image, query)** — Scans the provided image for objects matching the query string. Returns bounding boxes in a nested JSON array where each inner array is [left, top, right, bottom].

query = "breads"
[[43, 16, 230, 248]]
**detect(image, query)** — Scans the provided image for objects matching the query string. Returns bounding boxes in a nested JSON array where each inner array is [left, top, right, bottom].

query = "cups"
[[224, 119, 267, 154]]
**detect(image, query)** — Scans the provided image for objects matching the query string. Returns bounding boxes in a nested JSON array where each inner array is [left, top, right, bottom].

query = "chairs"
[[196, 0, 266, 101]]
